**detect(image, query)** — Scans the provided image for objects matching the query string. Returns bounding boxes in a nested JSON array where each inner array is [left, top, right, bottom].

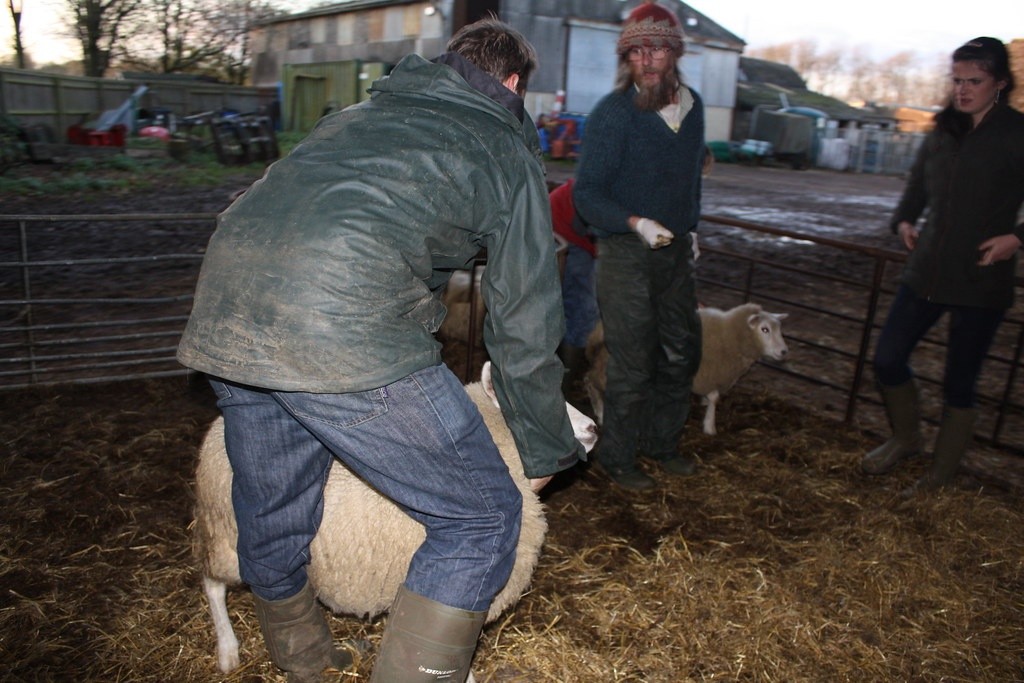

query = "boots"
[[859, 378, 924, 475], [641, 390, 696, 477], [371, 582, 489, 683], [250, 576, 368, 683], [598, 388, 654, 499], [913, 403, 978, 493]]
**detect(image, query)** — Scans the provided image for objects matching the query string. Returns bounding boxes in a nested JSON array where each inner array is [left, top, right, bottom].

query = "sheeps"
[[192, 361, 599, 683], [584, 302, 789, 435]]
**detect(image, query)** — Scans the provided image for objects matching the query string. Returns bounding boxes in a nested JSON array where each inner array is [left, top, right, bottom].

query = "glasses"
[[629, 45, 673, 61]]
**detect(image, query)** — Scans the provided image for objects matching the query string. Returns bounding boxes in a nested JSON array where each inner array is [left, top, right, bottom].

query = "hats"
[[616, 5, 685, 58]]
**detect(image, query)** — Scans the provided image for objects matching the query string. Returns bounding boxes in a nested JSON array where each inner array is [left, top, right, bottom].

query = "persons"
[[172, 17, 589, 682], [570, 0, 708, 494], [547, 145, 715, 416], [858, 35, 1024, 489]]
